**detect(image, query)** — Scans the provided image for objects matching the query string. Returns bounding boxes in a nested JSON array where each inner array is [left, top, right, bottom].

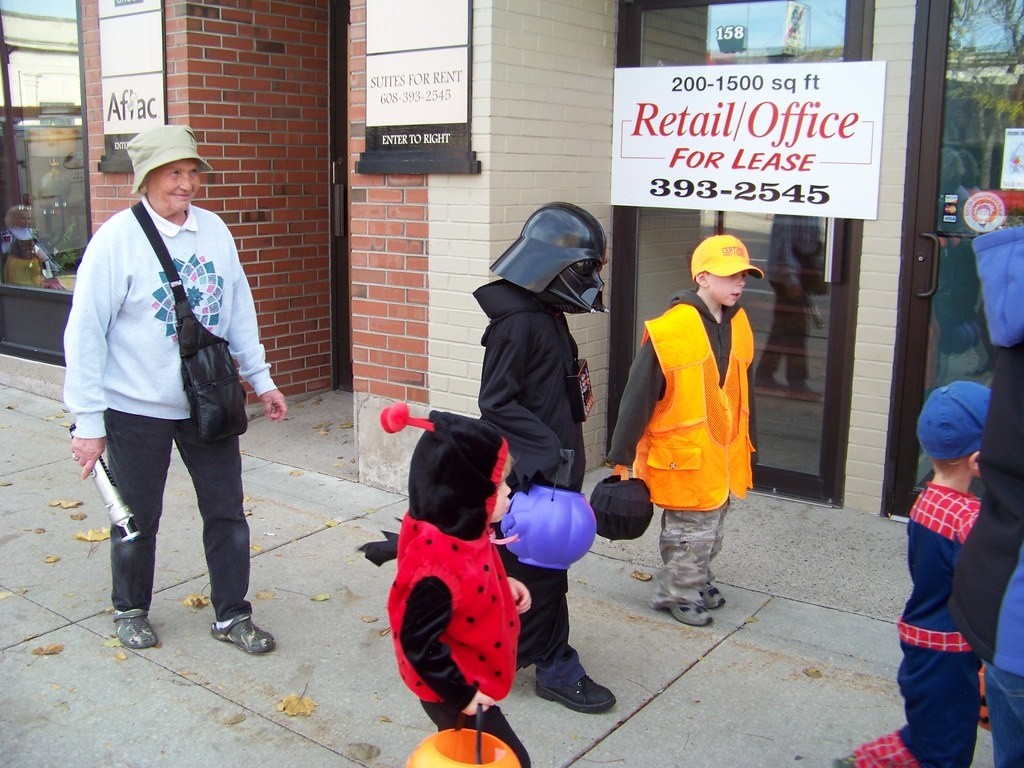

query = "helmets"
[[489, 202, 611, 314]]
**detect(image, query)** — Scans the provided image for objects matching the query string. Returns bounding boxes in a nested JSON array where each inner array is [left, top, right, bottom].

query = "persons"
[[947, 227, 1024, 768], [472, 202, 617, 713], [63, 124, 288, 655], [603, 234, 764, 628], [358, 403, 531, 767], [836, 382, 990, 768], [1, 205, 57, 288], [754, 212, 826, 403]]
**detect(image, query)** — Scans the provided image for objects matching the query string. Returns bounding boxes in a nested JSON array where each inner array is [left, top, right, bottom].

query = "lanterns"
[[404, 705, 522, 768], [499, 464, 597, 570], [589, 474, 655, 542], [977, 664, 990, 732]]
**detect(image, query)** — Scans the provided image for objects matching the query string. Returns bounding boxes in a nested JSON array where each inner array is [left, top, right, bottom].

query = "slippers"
[[113, 608, 158, 649], [211, 613, 276, 652]]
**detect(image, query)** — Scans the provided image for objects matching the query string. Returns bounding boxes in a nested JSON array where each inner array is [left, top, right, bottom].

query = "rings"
[[73, 452, 79, 460]]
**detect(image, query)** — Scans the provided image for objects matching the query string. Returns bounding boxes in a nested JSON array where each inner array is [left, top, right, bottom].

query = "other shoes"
[[756, 376, 784, 389], [792, 383, 821, 398]]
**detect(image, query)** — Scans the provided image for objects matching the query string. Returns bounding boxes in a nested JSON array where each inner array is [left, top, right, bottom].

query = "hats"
[[126, 124, 212, 193], [918, 382, 993, 460], [691, 234, 764, 283]]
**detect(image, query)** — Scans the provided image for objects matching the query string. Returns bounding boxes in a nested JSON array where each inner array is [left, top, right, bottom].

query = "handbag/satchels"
[[174, 299, 249, 443]]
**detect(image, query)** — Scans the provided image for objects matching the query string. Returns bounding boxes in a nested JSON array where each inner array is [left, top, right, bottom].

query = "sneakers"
[[650, 595, 713, 626], [536, 674, 616, 712], [700, 581, 726, 609]]
